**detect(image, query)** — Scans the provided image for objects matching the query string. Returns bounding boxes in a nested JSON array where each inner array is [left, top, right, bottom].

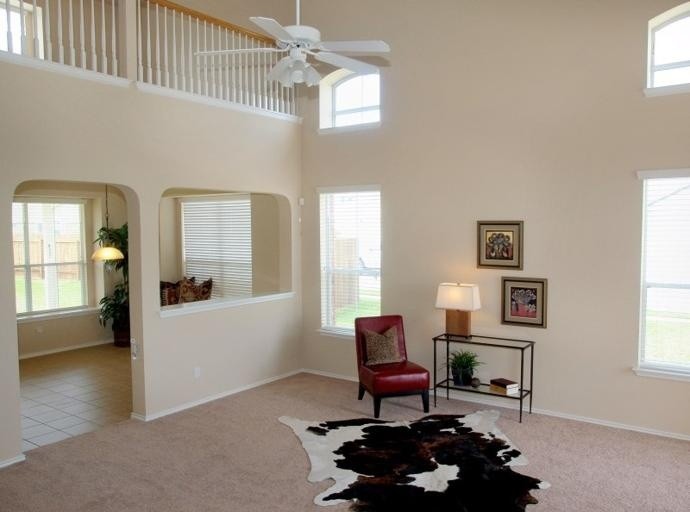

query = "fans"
[[190, 1, 391, 75]]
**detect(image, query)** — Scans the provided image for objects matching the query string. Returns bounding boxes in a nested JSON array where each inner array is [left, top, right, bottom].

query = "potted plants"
[[439, 350, 486, 386], [97, 225, 130, 348]]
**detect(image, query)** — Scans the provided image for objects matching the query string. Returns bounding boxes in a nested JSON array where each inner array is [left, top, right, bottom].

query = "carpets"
[[278, 409, 551, 512]]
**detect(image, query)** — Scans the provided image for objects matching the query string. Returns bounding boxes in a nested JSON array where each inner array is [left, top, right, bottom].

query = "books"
[[487, 377, 519, 396]]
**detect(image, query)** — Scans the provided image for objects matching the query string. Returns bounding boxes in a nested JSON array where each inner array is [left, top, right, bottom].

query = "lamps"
[[268, 53, 323, 89], [435, 283, 480, 339], [91, 183, 124, 262]]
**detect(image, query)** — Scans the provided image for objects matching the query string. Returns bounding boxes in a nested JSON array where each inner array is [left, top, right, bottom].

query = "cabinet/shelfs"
[[432, 332, 536, 423]]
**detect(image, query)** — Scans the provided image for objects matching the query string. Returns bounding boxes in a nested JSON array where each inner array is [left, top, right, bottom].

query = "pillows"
[[177, 278, 212, 303], [160, 276, 195, 306], [361, 325, 402, 366]]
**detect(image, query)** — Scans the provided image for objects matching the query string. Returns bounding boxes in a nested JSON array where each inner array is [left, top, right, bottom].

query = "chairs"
[[353, 316, 429, 418]]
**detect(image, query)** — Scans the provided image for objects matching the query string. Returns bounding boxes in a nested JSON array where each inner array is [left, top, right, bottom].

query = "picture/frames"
[[475, 219, 524, 270], [500, 276, 549, 330]]
